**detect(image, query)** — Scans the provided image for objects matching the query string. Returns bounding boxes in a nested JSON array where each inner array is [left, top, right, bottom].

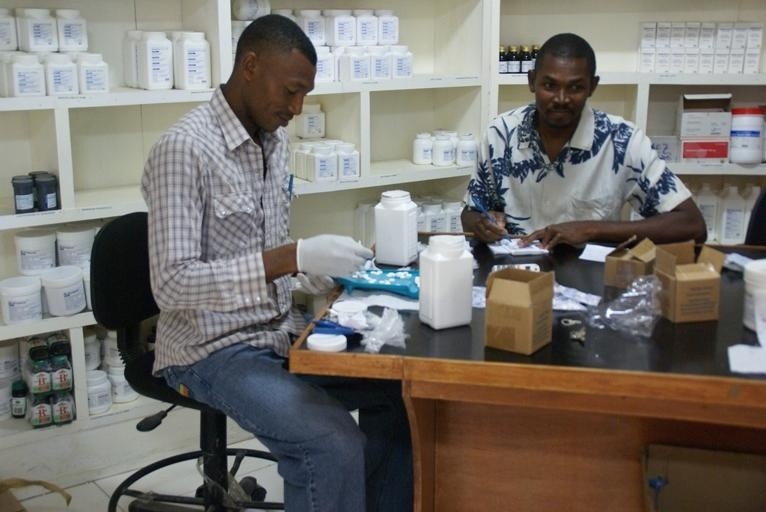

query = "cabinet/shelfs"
[[230, 6, 486, 268], [500, 2, 766, 252], [3, 2, 224, 432]]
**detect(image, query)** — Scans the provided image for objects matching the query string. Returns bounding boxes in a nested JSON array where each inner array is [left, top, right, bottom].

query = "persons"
[[459, 32, 708, 252], [138, 14, 416, 512]]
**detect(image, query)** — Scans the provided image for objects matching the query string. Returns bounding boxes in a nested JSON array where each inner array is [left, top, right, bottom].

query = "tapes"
[[305, 333, 346, 351]]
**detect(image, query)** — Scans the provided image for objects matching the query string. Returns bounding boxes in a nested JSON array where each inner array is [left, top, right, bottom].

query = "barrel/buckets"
[[727, 104, 766, 163], [0, 221, 92, 324]]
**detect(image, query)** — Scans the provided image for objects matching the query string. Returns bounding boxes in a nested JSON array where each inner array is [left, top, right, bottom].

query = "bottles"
[[10, 170, 61, 214], [410, 128, 478, 169], [499, 44, 539, 74], [0, 336, 76, 428], [373, 189, 419, 268], [231, 1, 415, 81], [418, 232, 475, 329], [740, 259, 766, 335], [83, 328, 140, 416], [288, 102, 361, 184], [0, 8, 213, 101]]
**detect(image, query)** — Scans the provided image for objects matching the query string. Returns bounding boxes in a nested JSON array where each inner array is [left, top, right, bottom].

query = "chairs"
[[89, 210, 285, 512]]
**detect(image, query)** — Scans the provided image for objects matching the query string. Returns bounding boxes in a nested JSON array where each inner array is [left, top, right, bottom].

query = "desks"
[[288, 230, 766, 512]]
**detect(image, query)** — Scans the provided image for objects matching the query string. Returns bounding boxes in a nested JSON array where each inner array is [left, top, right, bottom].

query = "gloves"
[[295, 234, 374, 296]]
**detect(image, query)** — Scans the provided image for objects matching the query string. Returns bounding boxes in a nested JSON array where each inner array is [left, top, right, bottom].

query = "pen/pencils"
[[471, 196, 513, 242]]
